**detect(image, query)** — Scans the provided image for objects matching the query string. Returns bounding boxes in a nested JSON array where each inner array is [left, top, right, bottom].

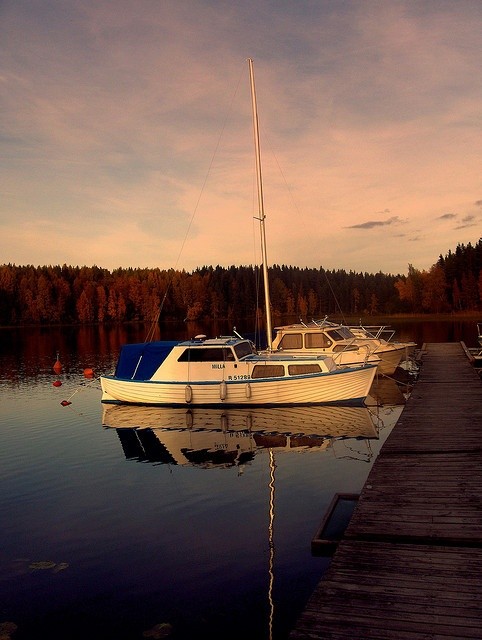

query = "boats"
[[102, 409, 379, 468], [349, 324, 417, 362], [102, 333, 381, 407]]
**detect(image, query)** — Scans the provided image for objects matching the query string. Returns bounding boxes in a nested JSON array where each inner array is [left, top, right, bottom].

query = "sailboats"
[[140, 55, 406, 378]]
